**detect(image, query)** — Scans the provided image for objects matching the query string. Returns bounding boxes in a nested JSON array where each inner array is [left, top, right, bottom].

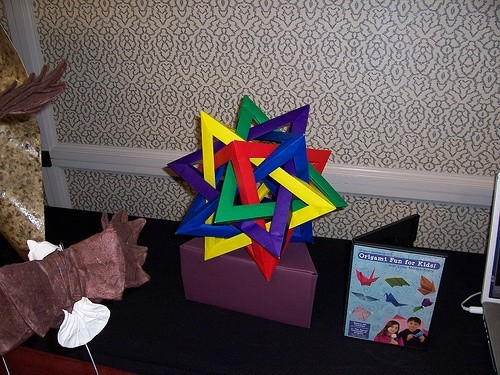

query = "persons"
[[374, 320, 404, 347], [398, 317, 428, 349]]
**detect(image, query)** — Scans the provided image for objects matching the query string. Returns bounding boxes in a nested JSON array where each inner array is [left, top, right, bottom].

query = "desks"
[[1, 204, 499, 375]]
